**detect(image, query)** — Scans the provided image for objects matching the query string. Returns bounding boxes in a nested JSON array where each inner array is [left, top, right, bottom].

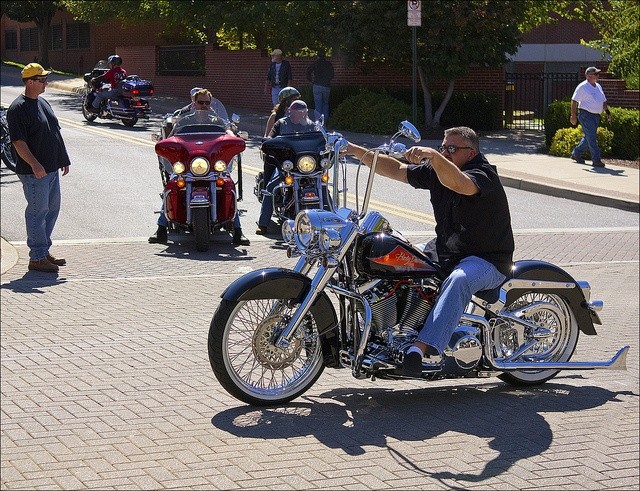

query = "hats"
[[585, 67, 602, 75], [20, 62, 52, 81], [271, 49, 283, 56]]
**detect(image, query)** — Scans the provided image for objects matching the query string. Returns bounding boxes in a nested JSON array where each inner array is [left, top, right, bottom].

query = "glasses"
[[195, 100, 211, 105], [590, 72, 600, 75], [30, 75, 48, 83], [436, 145, 475, 154]]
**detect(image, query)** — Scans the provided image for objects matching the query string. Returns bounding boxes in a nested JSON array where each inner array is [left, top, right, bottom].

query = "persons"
[[148, 88, 250, 247], [306, 50, 334, 124], [339, 125, 513, 376], [255, 100, 317, 236], [569, 66, 612, 169], [264, 49, 291, 108], [6, 63, 71, 274], [91, 55, 129, 111], [263, 86, 302, 138], [177, 86, 203, 116]]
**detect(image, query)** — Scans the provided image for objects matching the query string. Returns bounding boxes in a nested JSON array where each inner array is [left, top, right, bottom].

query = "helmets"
[[278, 86, 301, 106], [107, 54, 122, 64]]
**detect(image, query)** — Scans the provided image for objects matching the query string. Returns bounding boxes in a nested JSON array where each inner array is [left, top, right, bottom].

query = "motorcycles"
[[151, 110, 248, 252], [208, 114, 631, 408], [82, 60, 154, 127], [253, 112, 342, 228], [0, 105, 17, 170]]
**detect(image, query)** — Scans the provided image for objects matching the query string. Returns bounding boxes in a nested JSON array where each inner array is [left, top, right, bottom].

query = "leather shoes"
[[46, 254, 66, 265], [28, 257, 59, 272]]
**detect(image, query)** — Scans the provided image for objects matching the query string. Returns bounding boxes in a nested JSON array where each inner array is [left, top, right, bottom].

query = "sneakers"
[[233, 228, 250, 244], [571, 155, 585, 163], [593, 160, 605, 166], [402, 345, 424, 379], [256, 224, 267, 234], [148, 228, 167, 242]]
